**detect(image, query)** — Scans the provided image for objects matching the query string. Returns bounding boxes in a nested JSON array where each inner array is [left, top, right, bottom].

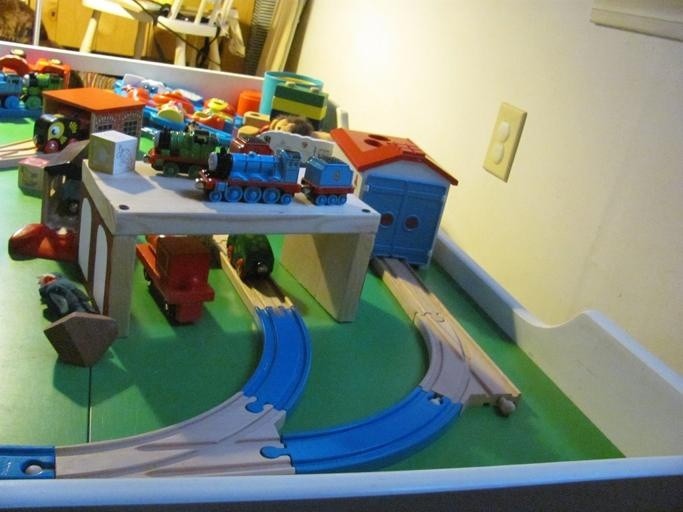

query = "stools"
[[74, 157, 382, 339]]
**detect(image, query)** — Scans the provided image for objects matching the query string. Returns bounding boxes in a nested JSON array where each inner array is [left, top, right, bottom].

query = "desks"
[[79, 1, 164, 60]]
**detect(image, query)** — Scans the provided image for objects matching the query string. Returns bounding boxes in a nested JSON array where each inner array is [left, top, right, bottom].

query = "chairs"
[[156, 0, 232, 72]]
